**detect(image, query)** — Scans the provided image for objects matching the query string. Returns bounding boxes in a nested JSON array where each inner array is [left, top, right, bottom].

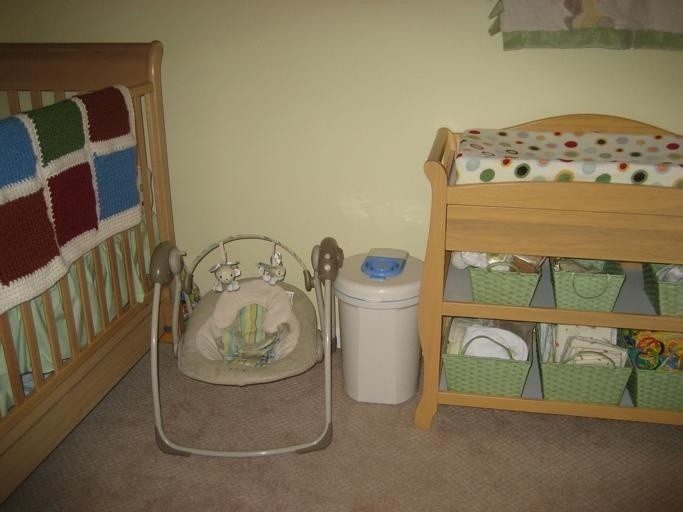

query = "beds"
[[0, 38, 188, 505]]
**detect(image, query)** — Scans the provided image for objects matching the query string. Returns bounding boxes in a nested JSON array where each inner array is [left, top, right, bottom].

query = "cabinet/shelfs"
[[412, 114, 683, 432]]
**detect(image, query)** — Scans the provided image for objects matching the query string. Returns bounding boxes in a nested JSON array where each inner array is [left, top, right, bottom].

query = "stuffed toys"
[[255, 252, 286, 286], [209, 258, 240, 293]]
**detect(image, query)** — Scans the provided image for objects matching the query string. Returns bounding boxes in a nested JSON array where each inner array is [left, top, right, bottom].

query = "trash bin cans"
[[332, 249, 424, 404]]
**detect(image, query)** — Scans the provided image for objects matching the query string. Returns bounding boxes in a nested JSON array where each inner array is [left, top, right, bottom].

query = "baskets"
[[441, 316, 533, 399], [536, 322, 634, 405], [644, 262, 683, 317], [620, 328, 683, 410], [549, 256, 626, 313], [467, 265, 543, 311]]
